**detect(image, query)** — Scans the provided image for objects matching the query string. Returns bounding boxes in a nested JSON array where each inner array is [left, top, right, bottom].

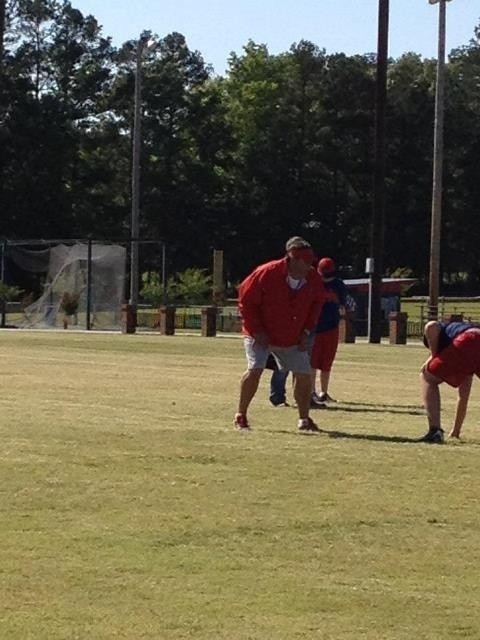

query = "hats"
[[316, 257, 336, 273]]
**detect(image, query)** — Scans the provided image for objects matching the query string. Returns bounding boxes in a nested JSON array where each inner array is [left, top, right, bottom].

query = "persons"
[[417, 319, 479, 444], [266, 352, 328, 409], [311, 257, 358, 403], [233, 234, 326, 434]]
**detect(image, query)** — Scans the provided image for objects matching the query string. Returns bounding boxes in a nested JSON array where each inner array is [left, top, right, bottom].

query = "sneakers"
[[271, 391, 337, 409], [298, 417, 319, 432], [234, 414, 251, 431], [413, 428, 444, 443]]
